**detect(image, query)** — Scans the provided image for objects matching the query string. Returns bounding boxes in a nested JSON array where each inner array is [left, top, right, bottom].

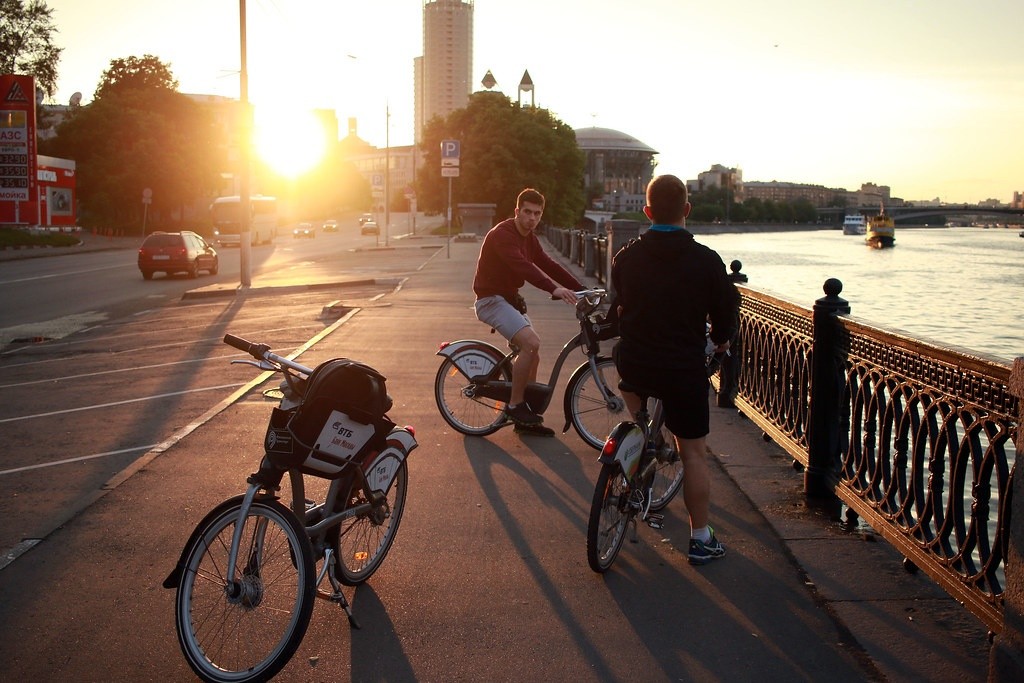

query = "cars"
[[363, 213, 373, 220], [294, 223, 314, 238], [361, 222, 376, 237], [322, 222, 339, 230], [137, 233, 219, 278]]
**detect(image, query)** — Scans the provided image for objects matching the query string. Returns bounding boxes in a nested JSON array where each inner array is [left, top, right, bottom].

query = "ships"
[[865, 203, 894, 250]]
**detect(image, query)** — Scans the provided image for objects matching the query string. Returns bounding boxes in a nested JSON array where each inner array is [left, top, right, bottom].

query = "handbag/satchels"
[[264, 359, 394, 480], [506, 290, 527, 314], [576, 294, 621, 342]]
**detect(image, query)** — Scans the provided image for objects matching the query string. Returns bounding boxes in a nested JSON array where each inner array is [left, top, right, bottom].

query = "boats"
[[843, 214, 864, 236]]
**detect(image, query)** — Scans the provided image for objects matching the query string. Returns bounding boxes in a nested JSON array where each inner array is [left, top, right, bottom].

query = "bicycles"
[[165, 334, 419, 683], [587, 324, 731, 573], [435, 296, 665, 455]]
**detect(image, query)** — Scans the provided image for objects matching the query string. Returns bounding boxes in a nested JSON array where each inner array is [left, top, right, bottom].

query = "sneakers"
[[514, 421, 555, 436], [688, 524, 724, 564], [504, 402, 543, 426]]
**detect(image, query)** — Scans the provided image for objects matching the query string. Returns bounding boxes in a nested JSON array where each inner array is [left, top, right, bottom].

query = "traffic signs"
[[440, 143, 460, 178]]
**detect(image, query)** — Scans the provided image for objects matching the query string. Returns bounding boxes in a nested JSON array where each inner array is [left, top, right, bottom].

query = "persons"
[[473, 189, 592, 437], [608, 174, 738, 560]]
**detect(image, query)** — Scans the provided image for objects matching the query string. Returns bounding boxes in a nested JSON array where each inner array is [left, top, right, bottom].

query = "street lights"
[[347, 55, 392, 247]]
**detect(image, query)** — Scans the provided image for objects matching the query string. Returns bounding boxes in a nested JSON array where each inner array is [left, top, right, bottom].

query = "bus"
[[213, 198, 282, 246]]
